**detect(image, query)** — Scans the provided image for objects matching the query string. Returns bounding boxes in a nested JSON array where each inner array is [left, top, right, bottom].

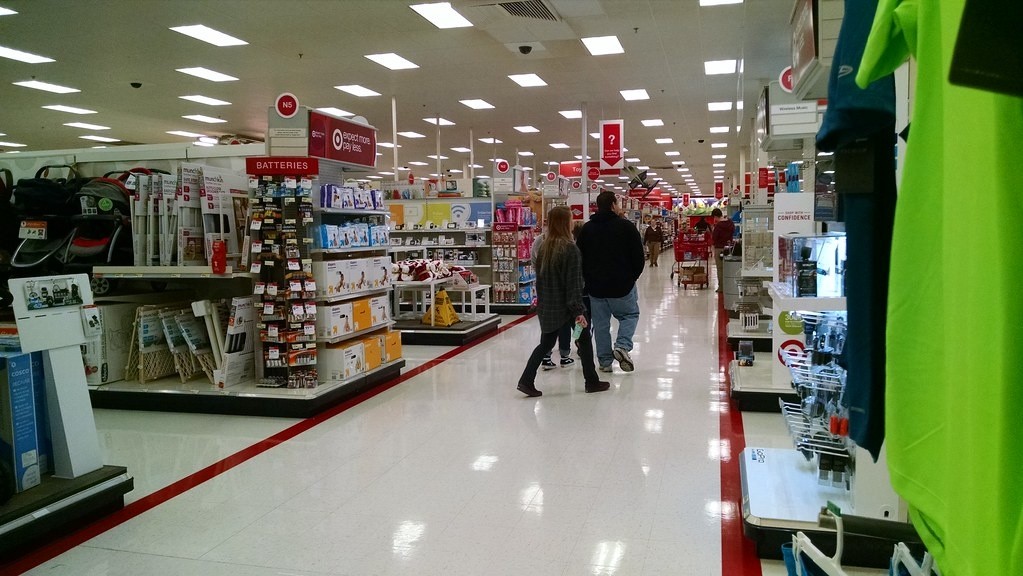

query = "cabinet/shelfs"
[[1, 143, 313, 417], [379, 173, 675, 344], [308, 154, 405, 401]]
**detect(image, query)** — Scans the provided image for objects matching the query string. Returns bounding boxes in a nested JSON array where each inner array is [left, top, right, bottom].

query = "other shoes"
[[517, 384, 542, 396], [654, 262, 658, 267], [575, 339, 580, 357], [585, 382, 610, 392], [649, 263, 653, 266]]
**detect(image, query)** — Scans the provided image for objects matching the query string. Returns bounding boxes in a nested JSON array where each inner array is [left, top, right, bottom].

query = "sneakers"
[[560, 358, 575, 368], [541, 359, 556, 369], [599, 363, 613, 372], [613, 348, 634, 372]]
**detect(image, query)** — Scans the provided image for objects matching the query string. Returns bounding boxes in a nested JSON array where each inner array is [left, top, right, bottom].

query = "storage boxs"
[[218, 296, 255, 389], [0, 332, 104, 495], [311, 255, 403, 383], [80, 300, 139, 386]]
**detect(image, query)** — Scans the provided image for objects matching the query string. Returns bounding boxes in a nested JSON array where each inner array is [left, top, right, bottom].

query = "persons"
[[644, 219, 664, 267], [577, 191, 645, 372], [694, 216, 711, 231], [711, 209, 735, 293], [517, 205, 610, 397]]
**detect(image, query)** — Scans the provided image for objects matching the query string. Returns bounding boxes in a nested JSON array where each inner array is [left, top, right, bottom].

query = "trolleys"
[[668, 228, 715, 291]]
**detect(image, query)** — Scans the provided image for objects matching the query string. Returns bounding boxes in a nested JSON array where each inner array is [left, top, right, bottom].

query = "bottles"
[[572, 323, 583, 339]]
